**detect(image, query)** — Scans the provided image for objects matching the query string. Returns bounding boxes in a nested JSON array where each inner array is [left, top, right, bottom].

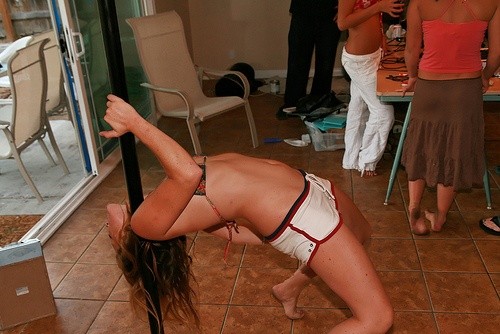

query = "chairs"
[[125, 8, 258, 155], [0, 38, 71, 203], [0, 28, 75, 167]]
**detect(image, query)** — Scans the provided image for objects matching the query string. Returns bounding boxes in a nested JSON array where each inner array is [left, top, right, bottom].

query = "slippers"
[[479, 215, 500, 236]]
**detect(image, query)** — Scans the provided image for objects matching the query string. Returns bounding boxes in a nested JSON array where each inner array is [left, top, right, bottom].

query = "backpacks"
[[290, 90, 345, 119]]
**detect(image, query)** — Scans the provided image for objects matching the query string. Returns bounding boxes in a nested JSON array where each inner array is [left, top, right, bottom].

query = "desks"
[[376, 36, 500, 209]]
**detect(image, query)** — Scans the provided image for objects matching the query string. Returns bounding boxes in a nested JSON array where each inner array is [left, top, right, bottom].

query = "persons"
[[404, 0, 500, 235], [99, 95, 394, 334], [337, 0, 404, 175], [276, 0, 349, 117]]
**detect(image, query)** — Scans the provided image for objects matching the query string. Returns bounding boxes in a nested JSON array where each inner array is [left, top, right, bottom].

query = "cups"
[[393, 25, 402, 37], [270, 80, 280, 93]]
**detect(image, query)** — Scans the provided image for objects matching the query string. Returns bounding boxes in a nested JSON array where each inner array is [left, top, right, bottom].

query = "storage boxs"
[[0, 239, 59, 331], [304, 120, 346, 152]]
